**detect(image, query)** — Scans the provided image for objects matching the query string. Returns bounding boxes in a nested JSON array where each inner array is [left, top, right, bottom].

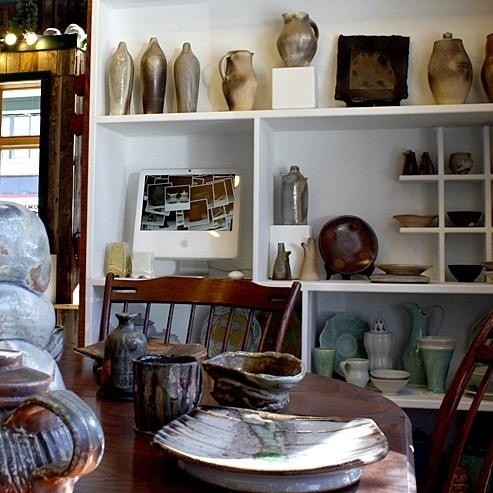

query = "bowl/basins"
[[448, 265, 483, 283], [369, 368, 410, 394], [448, 210, 484, 227]]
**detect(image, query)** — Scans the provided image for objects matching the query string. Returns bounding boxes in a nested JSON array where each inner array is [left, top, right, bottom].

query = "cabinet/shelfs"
[[83, 0, 493, 410]]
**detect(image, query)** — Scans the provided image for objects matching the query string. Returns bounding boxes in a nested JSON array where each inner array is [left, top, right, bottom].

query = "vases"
[[96, 9, 493, 395]]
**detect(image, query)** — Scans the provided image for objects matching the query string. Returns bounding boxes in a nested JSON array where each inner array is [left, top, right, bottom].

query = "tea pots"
[[1, 349, 107, 493], [399, 302, 445, 386], [277, 11, 320, 67], [219, 50, 257, 111]]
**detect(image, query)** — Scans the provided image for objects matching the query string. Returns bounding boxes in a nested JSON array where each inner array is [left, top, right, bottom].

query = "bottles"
[[107, 37, 201, 115], [101, 312, 150, 399], [280, 165, 309, 225]]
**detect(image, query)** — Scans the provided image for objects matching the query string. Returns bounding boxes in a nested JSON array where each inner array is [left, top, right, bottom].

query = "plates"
[[319, 313, 369, 359], [393, 213, 437, 229], [201, 308, 261, 358], [319, 214, 378, 275], [375, 264, 434, 276]]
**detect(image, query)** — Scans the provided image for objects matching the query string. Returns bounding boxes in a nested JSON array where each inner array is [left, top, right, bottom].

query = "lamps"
[[4, 31, 18, 46], [21, 29, 37, 46]]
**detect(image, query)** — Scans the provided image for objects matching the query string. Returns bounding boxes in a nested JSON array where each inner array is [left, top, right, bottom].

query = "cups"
[[136, 356, 203, 431], [311, 348, 336, 379], [339, 358, 369, 387], [416, 337, 459, 396]]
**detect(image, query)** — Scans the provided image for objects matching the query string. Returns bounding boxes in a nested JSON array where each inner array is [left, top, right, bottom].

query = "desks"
[[60, 350, 416, 493]]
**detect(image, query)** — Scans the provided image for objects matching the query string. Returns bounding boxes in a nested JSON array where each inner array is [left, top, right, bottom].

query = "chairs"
[[98, 272, 301, 353], [417, 311, 493, 493]]
